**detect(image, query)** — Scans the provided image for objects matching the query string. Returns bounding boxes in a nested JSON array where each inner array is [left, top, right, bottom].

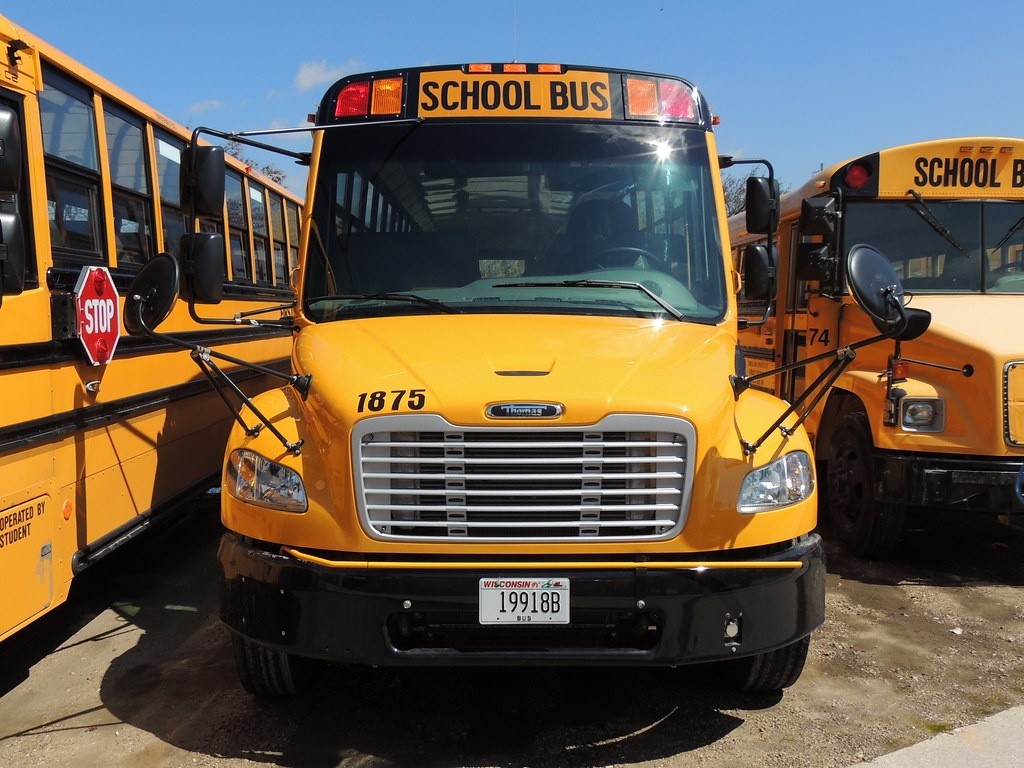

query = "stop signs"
[[73, 266, 124, 367]]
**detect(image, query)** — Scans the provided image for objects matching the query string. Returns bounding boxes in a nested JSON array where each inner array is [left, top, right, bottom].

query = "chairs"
[[943, 243, 992, 291], [328, 229, 486, 298], [564, 201, 641, 268]]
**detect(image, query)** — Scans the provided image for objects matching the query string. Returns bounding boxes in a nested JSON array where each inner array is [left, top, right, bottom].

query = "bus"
[[123, 63, 913, 728], [725, 138, 1024, 561], [0, 12, 307, 677]]
